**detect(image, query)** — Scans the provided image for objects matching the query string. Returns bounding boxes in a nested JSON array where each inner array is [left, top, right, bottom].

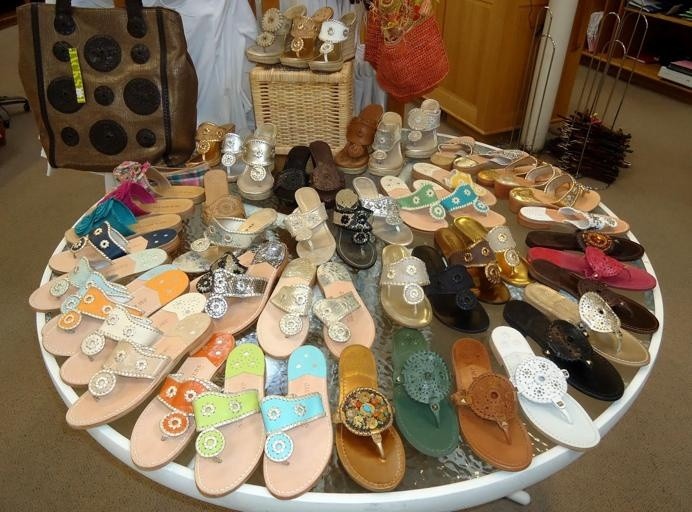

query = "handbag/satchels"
[[366, 7, 449, 97]]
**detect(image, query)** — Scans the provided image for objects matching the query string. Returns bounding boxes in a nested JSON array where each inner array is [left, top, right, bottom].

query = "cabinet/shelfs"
[[583, 2, 691, 106]]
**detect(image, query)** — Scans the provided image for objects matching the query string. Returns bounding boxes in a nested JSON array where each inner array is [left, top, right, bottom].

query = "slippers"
[[29, 158, 214, 430], [391, 328, 459, 458], [334, 104, 384, 176], [377, 148, 658, 334], [258, 344, 333, 502], [130, 331, 236, 471], [428, 134, 475, 167], [368, 112, 404, 177], [185, 121, 377, 358], [523, 281, 650, 366], [336, 342, 406, 492], [192, 344, 264, 497], [504, 301, 625, 401], [450, 339, 532, 473], [402, 98, 442, 160], [490, 325, 601, 451]]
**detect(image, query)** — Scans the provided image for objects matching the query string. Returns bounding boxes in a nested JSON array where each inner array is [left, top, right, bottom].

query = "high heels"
[[245, 4, 357, 70]]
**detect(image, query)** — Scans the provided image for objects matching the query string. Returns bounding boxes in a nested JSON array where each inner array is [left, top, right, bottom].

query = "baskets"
[[248, 58, 355, 156]]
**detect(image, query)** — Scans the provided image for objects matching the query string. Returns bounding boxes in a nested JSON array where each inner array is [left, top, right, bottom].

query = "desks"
[[35, 128, 665, 509]]
